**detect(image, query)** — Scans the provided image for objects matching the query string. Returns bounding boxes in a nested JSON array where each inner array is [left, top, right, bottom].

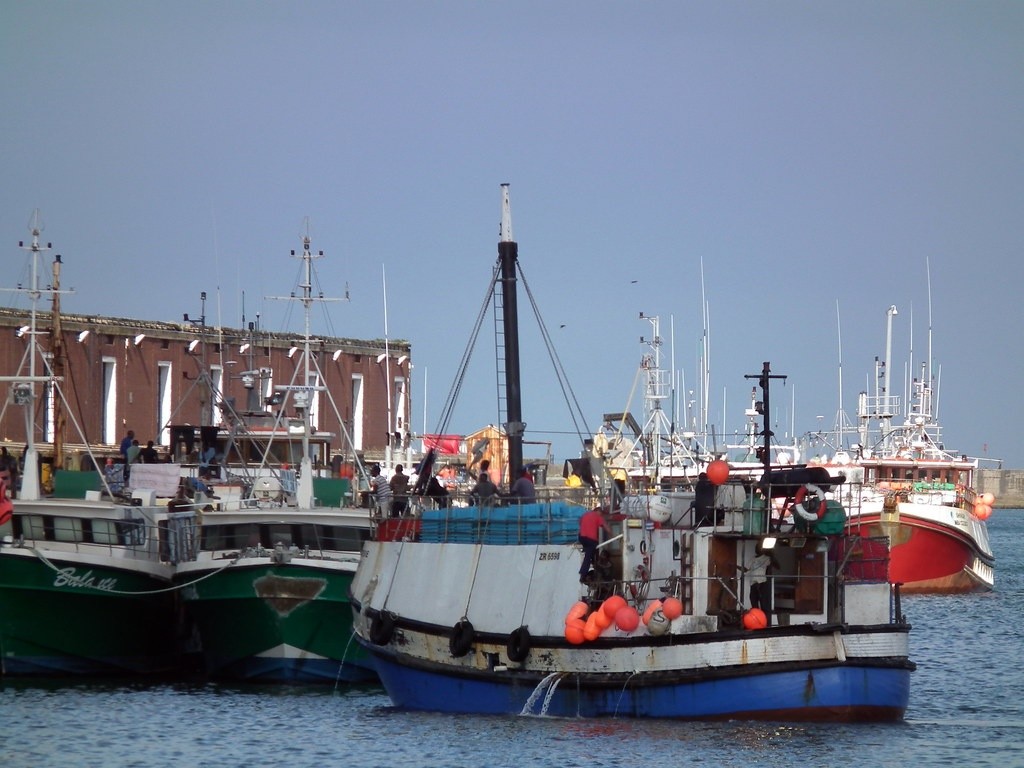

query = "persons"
[[120, 430, 158, 476], [509, 472, 536, 504], [578, 507, 616, 582], [389, 464, 411, 517], [370, 467, 393, 518], [565, 471, 582, 502], [202, 442, 220, 480], [694, 473, 716, 526], [750, 540, 796, 625], [419, 469, 453, 510], [469, 460, 506, 507], [0, 447, 18, 500], [611, 467, 626, 503]]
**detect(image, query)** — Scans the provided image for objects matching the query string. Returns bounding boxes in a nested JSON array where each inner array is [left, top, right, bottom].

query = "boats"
[[614, 254, 788, 497], [166, 232, 378, 692], [346, 183, 919, 732], [0, 208, 197, 678], [828, 259, 997, 595]]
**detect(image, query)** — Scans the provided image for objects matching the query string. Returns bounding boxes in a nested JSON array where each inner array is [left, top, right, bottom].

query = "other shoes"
[[580, 575, 593, 582]]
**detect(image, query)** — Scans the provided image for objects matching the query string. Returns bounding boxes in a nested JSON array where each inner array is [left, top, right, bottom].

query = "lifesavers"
[[506, 626, 531, 662], [630, 565, 651, 600], [449, 621, 475, 656], [794, 483, 827, 522], [369, 611, 396, 646]]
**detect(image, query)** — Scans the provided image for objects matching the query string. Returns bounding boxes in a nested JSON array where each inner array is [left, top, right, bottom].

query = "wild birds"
[[559, 324, 566, 328], [631, 280, 639, 283]]
